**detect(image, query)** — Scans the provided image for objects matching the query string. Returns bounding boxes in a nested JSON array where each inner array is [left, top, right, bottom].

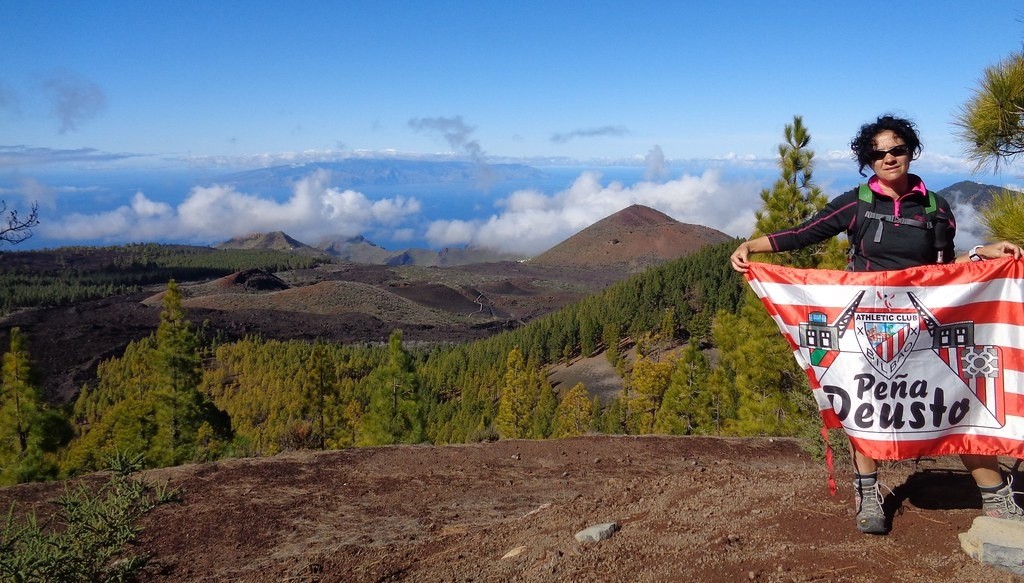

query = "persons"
[[729, 112, 1023, 537]]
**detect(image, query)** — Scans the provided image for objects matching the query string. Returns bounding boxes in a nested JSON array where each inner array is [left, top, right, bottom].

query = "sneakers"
[[982, 485, 1024, 522], [849, 478, 887, 533]]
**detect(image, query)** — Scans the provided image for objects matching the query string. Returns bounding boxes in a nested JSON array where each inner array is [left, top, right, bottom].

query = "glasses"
[[871, 143, 908, 162]]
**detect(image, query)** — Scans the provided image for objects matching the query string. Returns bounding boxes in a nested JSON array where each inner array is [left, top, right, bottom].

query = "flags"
[[739, 253, 1023, 495]]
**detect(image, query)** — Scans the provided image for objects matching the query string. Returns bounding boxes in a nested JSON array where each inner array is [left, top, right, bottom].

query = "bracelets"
[[968, 245, 987, 262]]
